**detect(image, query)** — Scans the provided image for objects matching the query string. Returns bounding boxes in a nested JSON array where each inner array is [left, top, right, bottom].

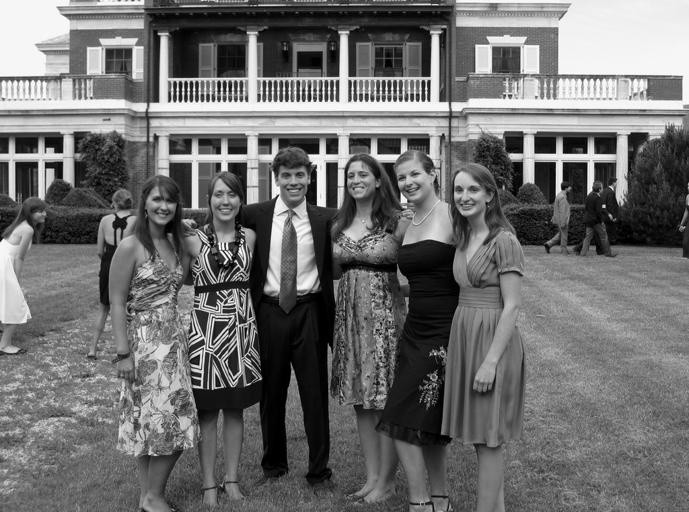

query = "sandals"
[[431, 494, 455, 512], [221, 479, 246, 502], [408, 501, 433, 511], [200, 485, 219, 509]]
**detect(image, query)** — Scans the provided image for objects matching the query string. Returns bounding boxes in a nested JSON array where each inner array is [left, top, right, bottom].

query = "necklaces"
[[206, 220, 241, 269], [412, 199, 442, 226], [355, 211, 371, 224]]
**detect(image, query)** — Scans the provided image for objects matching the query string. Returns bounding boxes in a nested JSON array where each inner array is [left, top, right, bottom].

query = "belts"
[[263, 293, 313, 306]]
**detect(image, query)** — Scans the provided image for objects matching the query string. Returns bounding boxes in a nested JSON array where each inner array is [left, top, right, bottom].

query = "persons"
[[678, 182, 689, 259], [183, 146, 416, 499], [85, 189, 138, 359], [573, 182, 604, 256], [375, 146, 462, 512], [0, 197, 47, 355], [601, 177, 618, 246], [108, 174, 194, 512], [331, 155, 408, 508], [543, 182, 572, 256], [126, 171, 265, 512], [440, 166, 526, 512], [579, 183, 618, 256]]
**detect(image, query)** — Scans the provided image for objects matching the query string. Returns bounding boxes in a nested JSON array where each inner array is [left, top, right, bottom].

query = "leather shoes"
[[253, 476, 279, 488], [543, 241, 550, 254], [0, 348, 27, 356]]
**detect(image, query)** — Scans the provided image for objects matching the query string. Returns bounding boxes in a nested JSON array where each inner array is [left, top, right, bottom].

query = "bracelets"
[[111, 352, 131, 365]]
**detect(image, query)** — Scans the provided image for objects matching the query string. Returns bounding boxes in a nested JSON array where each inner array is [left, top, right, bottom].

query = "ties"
[[278, 208, 298, 314]]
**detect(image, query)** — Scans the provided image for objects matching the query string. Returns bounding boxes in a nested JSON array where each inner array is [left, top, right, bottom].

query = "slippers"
[[85, 352, 96, 359]]
